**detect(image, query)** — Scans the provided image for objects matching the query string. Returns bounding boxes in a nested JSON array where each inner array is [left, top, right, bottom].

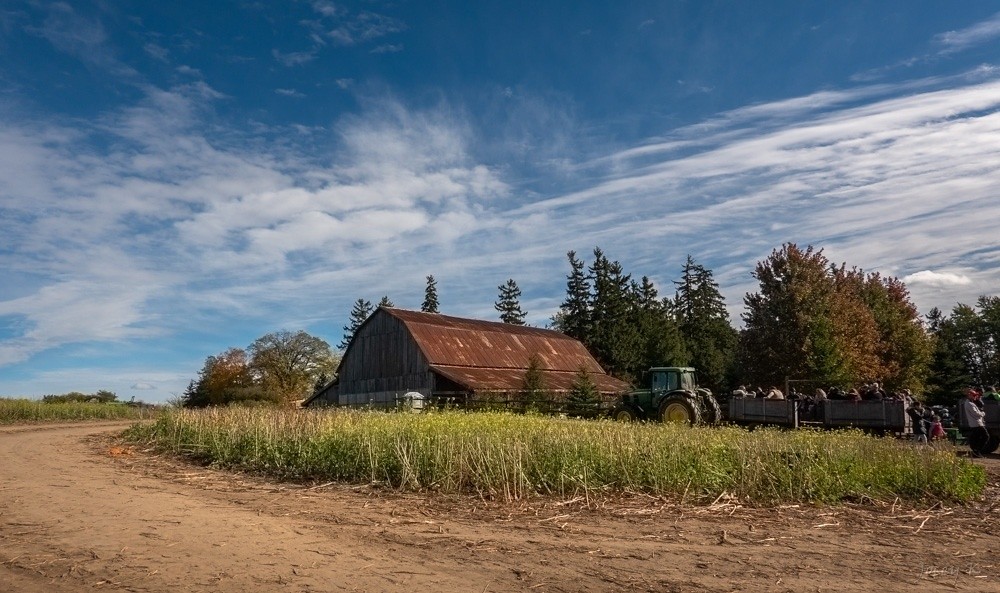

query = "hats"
[[968, 390, 979, 395], [873, 383, 879, 388]]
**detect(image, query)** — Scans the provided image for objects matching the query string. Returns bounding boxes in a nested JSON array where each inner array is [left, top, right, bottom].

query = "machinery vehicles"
[[606, 365, 722, 426]]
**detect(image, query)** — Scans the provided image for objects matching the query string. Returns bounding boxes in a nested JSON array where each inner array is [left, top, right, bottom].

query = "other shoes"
[[972, 450, 982, 457]]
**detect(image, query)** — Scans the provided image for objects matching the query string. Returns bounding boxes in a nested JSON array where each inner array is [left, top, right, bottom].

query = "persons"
[[788, 383, 914, 401], [907, 401, 951, 442], [734, 384, 784, 400], [958, 385, 1000, 457]]
